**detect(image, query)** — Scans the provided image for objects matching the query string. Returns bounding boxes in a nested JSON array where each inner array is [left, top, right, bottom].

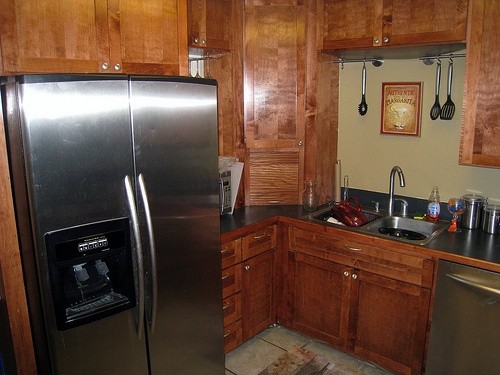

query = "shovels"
[[440, 63, 455, 120]]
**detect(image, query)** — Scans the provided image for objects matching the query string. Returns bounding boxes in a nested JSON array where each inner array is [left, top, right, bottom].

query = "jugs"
[[331, 195, 369, 227]]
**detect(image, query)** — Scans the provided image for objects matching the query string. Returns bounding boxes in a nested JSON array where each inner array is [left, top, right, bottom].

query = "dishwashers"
[[422, 259, 500, 375]]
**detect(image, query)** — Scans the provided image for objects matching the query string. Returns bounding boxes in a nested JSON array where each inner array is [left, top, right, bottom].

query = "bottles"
[[426, 186, 441, 223], [302, 179, 319, 212], [343, 175, 349, 203]]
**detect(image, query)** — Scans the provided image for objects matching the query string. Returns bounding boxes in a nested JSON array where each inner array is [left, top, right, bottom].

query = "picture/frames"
[[380, 82, 422, 137]]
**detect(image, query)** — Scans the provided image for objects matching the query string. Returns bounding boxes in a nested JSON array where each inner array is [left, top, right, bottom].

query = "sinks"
[[363, 215, 452, 246], [304, 203, 387, 231], [388, 165, 405, 214]]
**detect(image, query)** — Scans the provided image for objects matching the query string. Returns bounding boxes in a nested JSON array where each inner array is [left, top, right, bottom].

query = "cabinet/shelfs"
[[221, 221, 434, 375], [0, 0, 500, 205]]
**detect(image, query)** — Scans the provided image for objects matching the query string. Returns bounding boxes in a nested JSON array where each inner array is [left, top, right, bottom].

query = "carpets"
[[258, 344, 369, 375]]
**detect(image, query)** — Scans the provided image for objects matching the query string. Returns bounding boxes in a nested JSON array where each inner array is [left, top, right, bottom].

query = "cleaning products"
[[426, 186, 441, 223]]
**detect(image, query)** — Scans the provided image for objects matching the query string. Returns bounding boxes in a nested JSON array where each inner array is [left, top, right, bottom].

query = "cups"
[[461, 194, 488, 230], [480, 204, 500, 235]]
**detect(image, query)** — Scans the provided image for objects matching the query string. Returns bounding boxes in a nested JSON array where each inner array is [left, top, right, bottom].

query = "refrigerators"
[[0, 72, 227, 375]]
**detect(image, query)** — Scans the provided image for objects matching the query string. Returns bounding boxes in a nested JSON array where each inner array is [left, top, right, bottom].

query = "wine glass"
[[448, 197, 465, 233]]
